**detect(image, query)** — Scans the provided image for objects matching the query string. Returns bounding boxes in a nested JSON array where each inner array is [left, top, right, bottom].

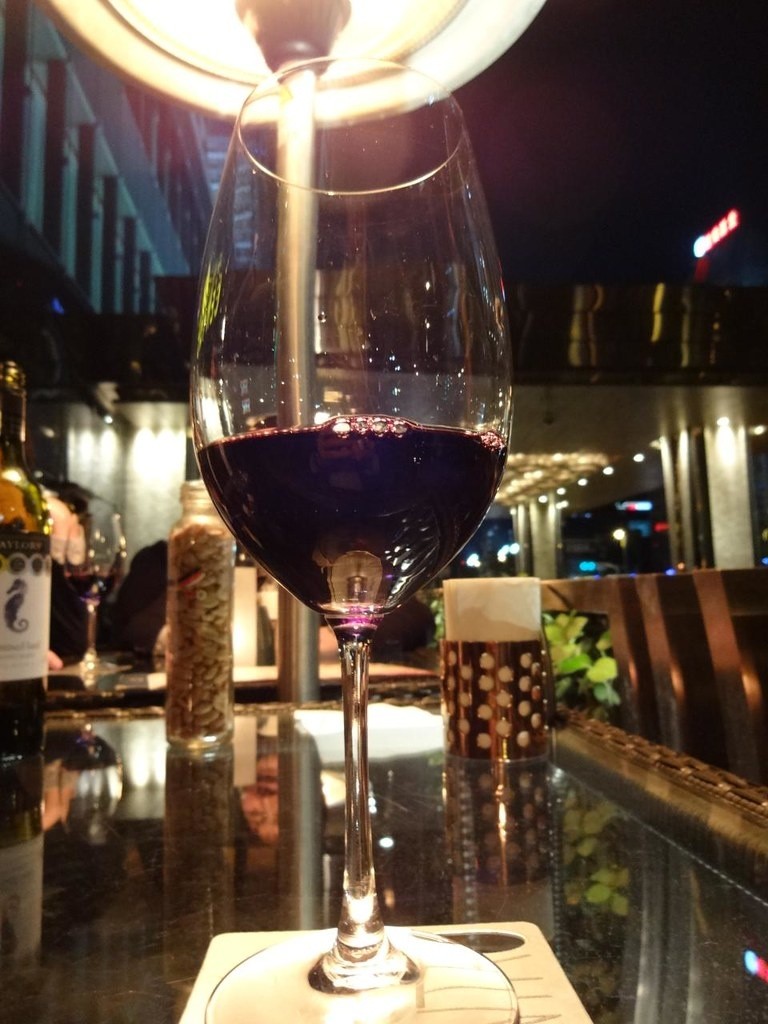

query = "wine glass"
[[189, 54, 519, 1024], [65, 510, 131, 677]]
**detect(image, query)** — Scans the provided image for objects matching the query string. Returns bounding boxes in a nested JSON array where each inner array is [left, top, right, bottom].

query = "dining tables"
[[0, 685, 768, 1024]]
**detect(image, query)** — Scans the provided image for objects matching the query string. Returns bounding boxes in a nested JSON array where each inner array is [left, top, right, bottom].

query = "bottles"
[[0, 362, 51, 762], [167, 478, 236, 747]]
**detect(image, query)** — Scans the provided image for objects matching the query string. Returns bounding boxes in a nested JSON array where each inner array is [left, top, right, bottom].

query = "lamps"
[[53, 0, 552, 704]]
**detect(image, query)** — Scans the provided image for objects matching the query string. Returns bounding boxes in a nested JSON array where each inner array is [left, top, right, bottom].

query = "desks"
[[47, 658, 444, 698]]
